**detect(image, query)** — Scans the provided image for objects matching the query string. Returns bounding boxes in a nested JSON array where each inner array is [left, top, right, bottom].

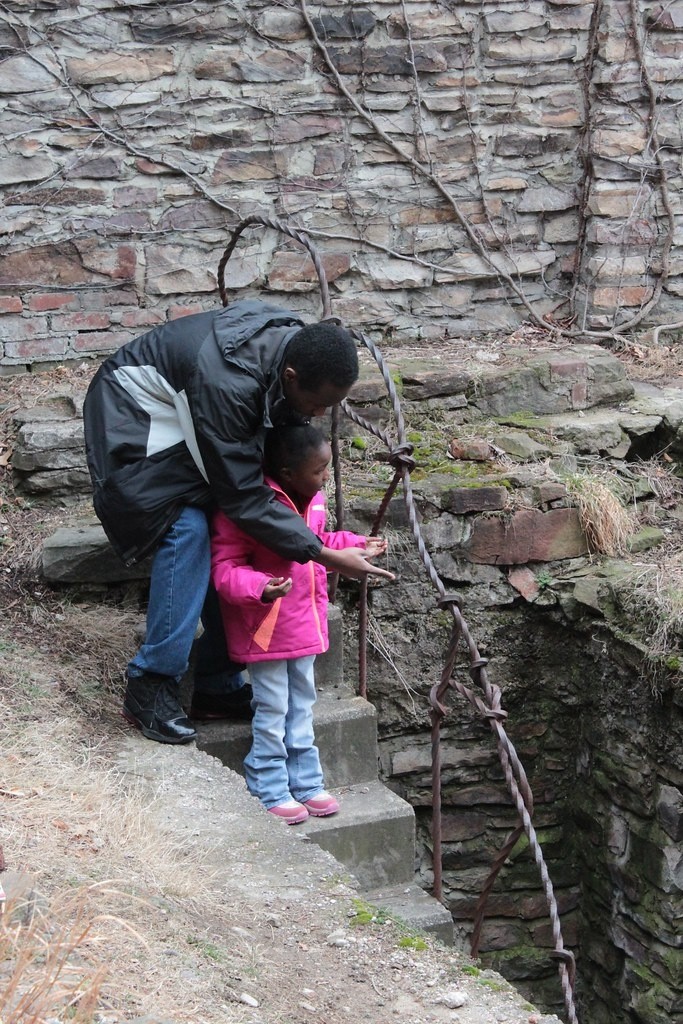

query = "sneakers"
[[255, 785, 339, 826], [190, 670, 254, 723], [122, 673, 199, 744]]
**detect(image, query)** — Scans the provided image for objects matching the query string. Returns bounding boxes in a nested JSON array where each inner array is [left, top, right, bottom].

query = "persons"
[[210, 409, 389, 825], [82, 300, 396, 745]]
